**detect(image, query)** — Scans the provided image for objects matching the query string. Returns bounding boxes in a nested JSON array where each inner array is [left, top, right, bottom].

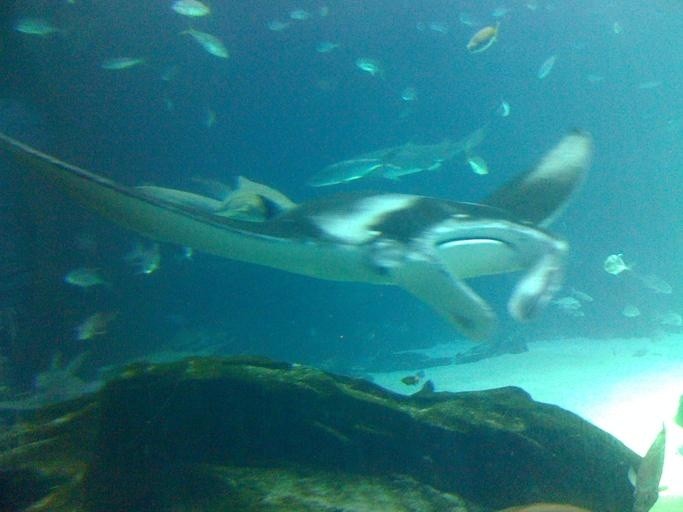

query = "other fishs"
[[0, 0, 683, 388]]
